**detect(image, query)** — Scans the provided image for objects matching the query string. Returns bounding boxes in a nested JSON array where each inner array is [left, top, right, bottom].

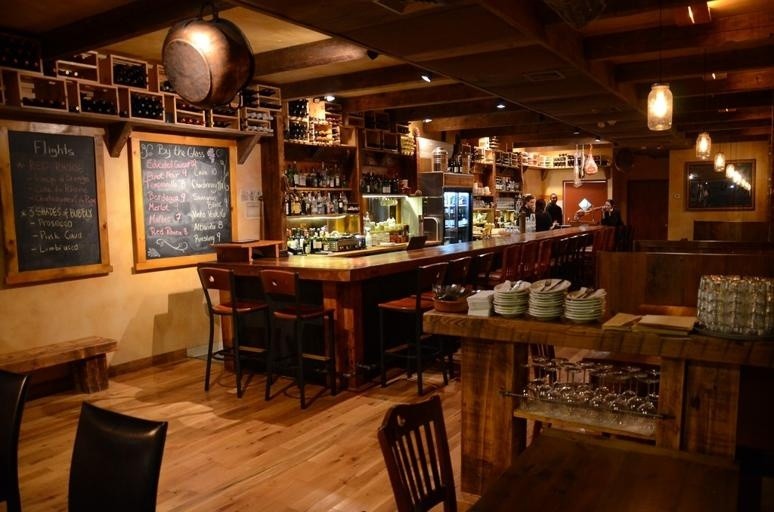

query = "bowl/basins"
[[432, 294, 468, 314]]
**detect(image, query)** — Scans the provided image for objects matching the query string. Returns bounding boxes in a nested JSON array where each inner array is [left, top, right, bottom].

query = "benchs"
[[595, 219, 774, 321], [0, 335, 118, 395]]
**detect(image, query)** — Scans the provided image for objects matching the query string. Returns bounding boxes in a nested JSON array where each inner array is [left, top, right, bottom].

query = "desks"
[[463, 428, 741, 512]]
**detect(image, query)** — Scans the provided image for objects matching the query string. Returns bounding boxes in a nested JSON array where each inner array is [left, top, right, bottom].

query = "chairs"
[[66, 401, 168, 512], [260, 268, 338, 412], [197, 266, 284, 399], [452, 224, 622, 293], [376, 394, 459, 512], [377, 259, 450, 394], [0, 369, 29, 511]]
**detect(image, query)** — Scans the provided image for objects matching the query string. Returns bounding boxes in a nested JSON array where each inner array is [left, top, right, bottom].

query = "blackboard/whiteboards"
[[0, 117, 113, 286], [127, 131, 240, 273]]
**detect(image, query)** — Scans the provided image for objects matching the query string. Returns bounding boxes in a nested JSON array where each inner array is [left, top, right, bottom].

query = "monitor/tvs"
[[361, 129, 382, 149], [426, 196, 444, 216], [365, 111, 391, 129], [382, 132, 398, 151], [418, 170, 444, 196]]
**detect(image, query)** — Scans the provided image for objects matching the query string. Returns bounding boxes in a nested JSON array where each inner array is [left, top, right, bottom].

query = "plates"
[[493, 282, 530, 319], [528, 278, 571, 321], [564, 291, 607, 326]]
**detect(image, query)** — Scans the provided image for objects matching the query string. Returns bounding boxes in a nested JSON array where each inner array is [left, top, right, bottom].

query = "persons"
[[533, 199, 560, 231], [547, 193, 563, 226], [600, 200, 623, 242], [518, 196, 534, 225]]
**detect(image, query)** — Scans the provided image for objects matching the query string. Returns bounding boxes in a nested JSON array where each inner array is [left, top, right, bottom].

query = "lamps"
[[710, 150, 727, 173], [420, 70, 434, 83], [646, 76, 674, 133], [496, 99, 507, 110], [366, 49, 380, 61], [694, 127, 714, 161], [726, 163, 751, 191]]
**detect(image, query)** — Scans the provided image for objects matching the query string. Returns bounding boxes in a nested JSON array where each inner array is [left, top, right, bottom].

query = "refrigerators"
[[418, 171, 475, 245]]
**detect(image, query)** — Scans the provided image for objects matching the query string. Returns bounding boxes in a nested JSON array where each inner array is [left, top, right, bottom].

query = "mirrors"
[[684, 159, 757, 213]]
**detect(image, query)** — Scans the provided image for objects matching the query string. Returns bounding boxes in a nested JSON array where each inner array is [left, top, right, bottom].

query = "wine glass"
[[520, 354, 660, 436]]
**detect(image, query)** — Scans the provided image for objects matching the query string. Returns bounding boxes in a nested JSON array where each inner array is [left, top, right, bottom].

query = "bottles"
[[259, 88, 275, 96], [364, 218, 410, 246], [213, 107, 235, 116], [359, 172, 399, 193], [399, 128, 407, 133], [247, 125, 274, 133], [284, 189, 349, 215], [260, 102, 282, 109], [213, 120, 232, 127], [286, 160, 348, 189], [160, 85, 176, 92], [286, 224, 329, 254], [246, 111, 274, 120], [326, 117, 340, 122], [5, 57, 41, 70], [114, 65, 146, 86], [176, 101, 200, 112], [291, 99, 309, 117], [132, 93, 163, 117], [178, 117, 200, 124], [70, 105, 80, 113], [58, 69, 79, 77], [44, 67, 57, 76], [120, 110, 128, 116], [291, 123, 307, 140], [22, 96, 65, 108], [448, 155, 463, 172], [316, 130, 335, 142], [243, 88, 260, 108], [84, 98, 117, 115]]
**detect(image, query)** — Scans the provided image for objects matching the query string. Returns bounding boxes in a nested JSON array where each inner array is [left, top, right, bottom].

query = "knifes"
[[549, 279, 569, 290], [583, 286, 600, 299]]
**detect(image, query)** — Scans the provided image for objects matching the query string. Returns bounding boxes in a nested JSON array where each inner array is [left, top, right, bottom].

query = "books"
[[600, 310, 697, 337]]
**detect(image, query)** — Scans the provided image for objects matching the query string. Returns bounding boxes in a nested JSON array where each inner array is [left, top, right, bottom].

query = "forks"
[[541, 280, 552, 293], [577, 287, 592, 298]]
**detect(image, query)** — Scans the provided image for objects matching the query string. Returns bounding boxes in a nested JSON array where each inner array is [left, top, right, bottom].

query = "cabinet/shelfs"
[[209, 240, 284, 374], [268, 136, 360, 252], [460, 148, 494, 236], [283, 94, 412, 151], [361, 147, 418, 199], [0, 40, 283, 134], [495, 150, 525, 231], [423, 288, 774, 494]]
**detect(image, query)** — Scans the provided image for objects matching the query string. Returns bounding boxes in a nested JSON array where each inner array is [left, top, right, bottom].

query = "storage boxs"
[[524, 151, 602, 168]]
